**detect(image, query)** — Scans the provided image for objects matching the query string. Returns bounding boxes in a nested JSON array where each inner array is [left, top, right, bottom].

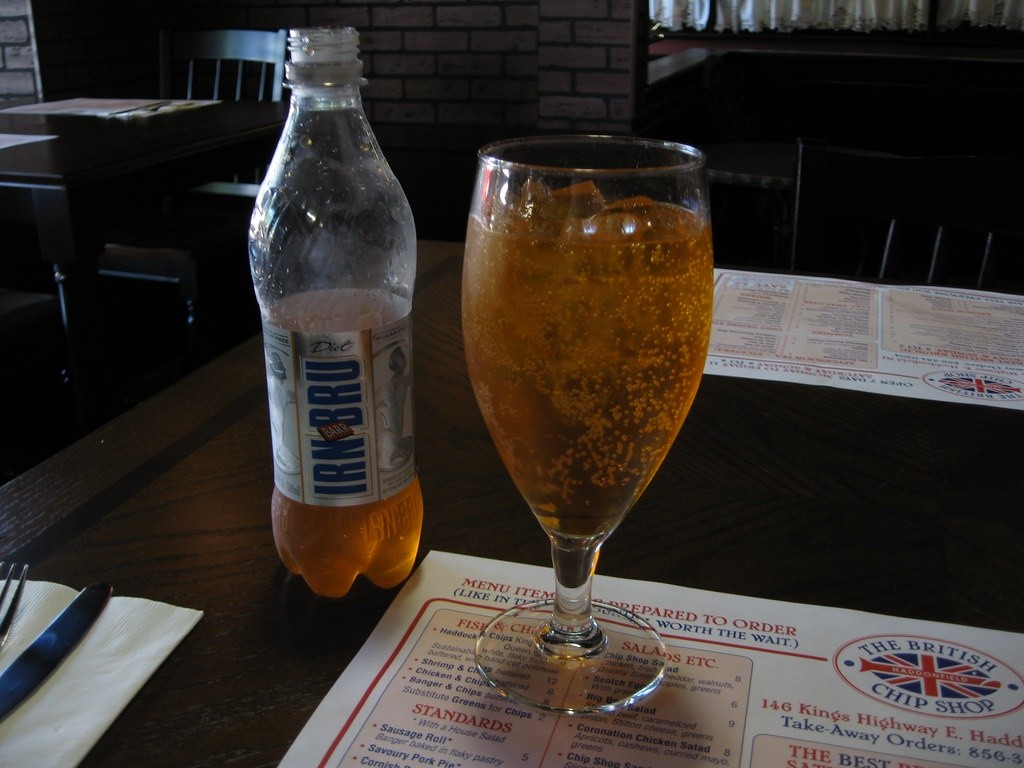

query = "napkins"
[[0, 574, 208, 768]]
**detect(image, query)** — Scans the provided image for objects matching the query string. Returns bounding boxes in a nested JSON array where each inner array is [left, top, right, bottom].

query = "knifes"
[[0, 583, 115, 730]]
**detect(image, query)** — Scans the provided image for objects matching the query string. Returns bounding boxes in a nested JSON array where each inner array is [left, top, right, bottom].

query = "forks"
[[0, 561, 29, 646]]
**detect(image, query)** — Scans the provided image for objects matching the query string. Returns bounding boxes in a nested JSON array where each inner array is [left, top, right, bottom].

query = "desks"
[[0, 239, 1024, 768], [0, 95, 287, 356]]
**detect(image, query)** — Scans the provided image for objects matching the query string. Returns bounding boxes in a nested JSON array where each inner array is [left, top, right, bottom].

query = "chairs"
[[156, 24, 287, 185], [781, 136, 1006, 288]]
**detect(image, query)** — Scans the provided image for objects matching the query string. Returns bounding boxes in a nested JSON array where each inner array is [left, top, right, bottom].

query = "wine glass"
[[460, 135, 716, 715]]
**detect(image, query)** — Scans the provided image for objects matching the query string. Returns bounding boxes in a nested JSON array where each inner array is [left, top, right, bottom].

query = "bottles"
[[247, 23, 425, 598]]
